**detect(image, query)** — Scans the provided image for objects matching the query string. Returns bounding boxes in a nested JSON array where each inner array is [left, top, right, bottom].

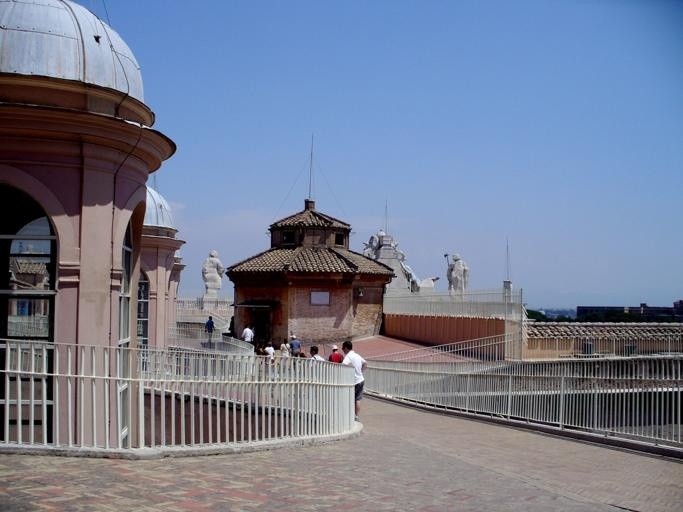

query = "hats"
[[290, 335, 296, 338], [331, 344, 338, 350]]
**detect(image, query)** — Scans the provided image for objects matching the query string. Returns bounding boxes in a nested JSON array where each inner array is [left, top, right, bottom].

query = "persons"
[[254, 332, 307, 363], [309, 346, 324, 367], [229, 316, 234, 333], [447, 254, 469, 294], [328, 345, 343, 363], [341, 341, 368, 422], [205, 316, 216, 342], [202, 250, 225, 290], [239, 325, 254, 344]]
